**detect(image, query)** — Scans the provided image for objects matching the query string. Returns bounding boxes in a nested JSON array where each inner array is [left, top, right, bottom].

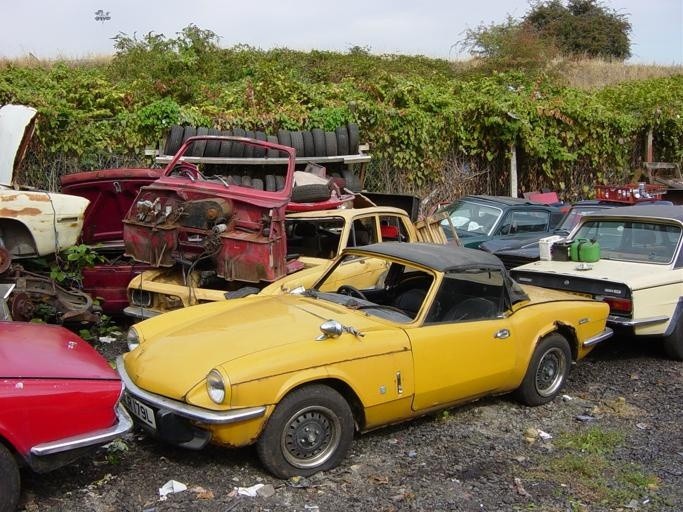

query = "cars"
[[0, 104, 423, 323], [492, 211, 610, 256], [1, 315, 133, 506], [118, 247, 606, 444], [514, 208, 683, 344]]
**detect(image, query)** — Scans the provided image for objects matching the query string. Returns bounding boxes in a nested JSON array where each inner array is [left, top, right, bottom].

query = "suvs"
[[438, 198, 549, 249]]
